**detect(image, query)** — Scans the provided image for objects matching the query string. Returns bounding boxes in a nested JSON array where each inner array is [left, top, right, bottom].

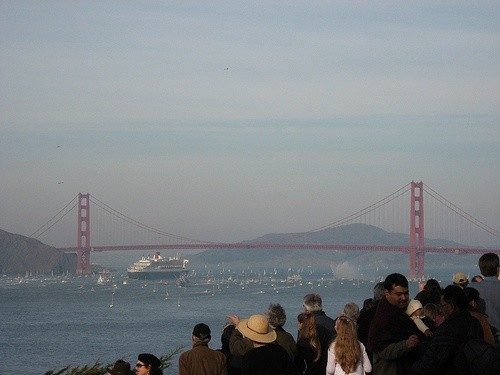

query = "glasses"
[[136, 364, 146, 368], [438, 302, 448, 307]]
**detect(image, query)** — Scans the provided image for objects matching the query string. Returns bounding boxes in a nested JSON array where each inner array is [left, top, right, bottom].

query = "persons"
[[106, 252, 500, 375]]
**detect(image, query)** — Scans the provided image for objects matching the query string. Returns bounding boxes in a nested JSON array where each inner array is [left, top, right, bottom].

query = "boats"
[[127, 252, 190, 281]]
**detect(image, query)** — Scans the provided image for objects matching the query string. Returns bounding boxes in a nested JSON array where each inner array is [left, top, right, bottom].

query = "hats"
[[192, 323, 211, 339], [107, 360, 137, 375], [138, 354, 162, 366], [236, 314, 277, 343], [452, 272, 467, 283], [404, 300, 423, 317]]
